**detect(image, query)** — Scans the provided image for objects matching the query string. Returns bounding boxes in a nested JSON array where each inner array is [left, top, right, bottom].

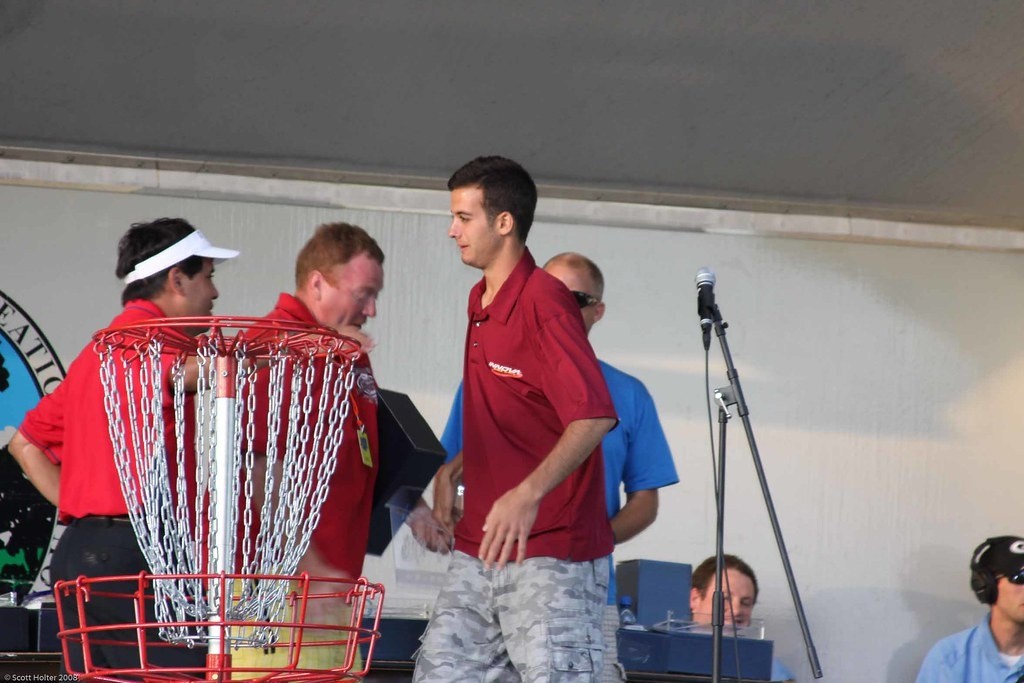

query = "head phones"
[[971, 535, 1024, 604]]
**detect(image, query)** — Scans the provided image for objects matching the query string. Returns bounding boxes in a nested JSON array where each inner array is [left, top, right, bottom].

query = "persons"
[[168, 224, 454, 683], [8, 217, 376, 683], [687, 555, 798, 683], [412, 155, 620, 683], [432, 252, 680, 683], [915, 536, 1024, 683]]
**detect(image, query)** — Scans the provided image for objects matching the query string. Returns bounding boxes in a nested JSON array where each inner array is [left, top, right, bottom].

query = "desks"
[[0, 650, 775, 683]]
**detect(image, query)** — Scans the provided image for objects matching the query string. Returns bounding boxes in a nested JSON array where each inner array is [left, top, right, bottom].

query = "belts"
[[74, 514, 132, 524]]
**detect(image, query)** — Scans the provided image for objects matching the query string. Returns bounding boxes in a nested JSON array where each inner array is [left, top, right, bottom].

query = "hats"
[[124, 229, 240, 285], [972, 536, 1024, 575]]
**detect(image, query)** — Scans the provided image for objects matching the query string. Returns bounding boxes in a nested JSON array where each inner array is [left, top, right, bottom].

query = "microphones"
[[694, 265, 717, 350]]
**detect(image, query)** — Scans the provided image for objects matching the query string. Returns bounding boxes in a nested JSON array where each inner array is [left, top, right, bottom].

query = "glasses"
[[571, 290, 601, 308], [994, 572, 1024, 585]]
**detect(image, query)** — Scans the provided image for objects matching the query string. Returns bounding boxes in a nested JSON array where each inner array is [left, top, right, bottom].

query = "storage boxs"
[[0, 602, 64, 652], [616, 627, 774, 681], [366, 388, 447, 557], [616, 559, 692, 631]]
